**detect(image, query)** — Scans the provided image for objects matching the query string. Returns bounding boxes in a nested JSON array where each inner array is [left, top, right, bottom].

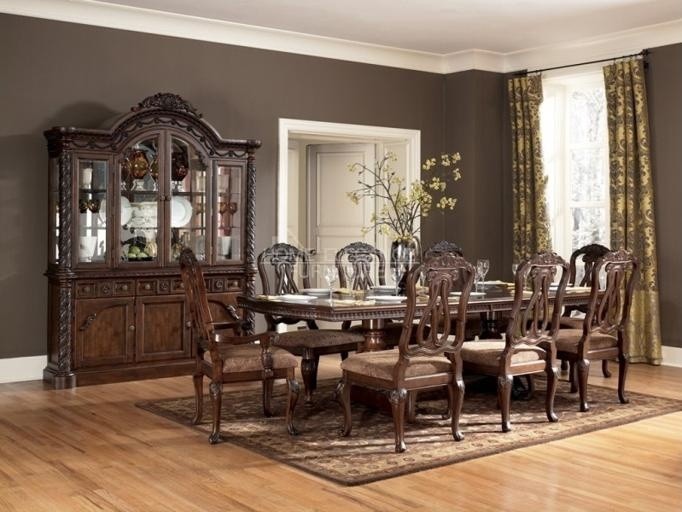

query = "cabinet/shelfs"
[[38, 89, 262, 390]]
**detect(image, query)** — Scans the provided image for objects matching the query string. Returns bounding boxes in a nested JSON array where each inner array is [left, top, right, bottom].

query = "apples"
[[128, 246, 147, 257]]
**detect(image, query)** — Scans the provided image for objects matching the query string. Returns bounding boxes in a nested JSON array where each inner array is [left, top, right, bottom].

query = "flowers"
[[338, 145, 465, 239]]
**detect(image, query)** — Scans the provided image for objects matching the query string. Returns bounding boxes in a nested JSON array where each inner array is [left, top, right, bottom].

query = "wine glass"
[[471, 257, 489, 293], [512, 263, 520, 275], [324, 267, 339, 303], [523, 264, 532, 290]]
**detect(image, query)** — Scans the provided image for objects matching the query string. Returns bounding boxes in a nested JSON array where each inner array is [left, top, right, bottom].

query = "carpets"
[[133, 363, 682, 487]]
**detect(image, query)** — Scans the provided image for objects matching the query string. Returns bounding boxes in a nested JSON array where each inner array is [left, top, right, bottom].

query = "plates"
[[299, 287, 331, 296], [547, 283, 573, 286], [99, 196, 133, 227], [478, 280, 507, 286], [370, 285, 401, 293], [366, 296, 408, 304], [171, 196, 193, 228], [124, 257, 152, 261], [278, 294, 319, 301], [450, 290, 487, 298]]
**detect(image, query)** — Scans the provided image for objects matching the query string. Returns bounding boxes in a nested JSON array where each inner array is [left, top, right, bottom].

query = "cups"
[[80, 236, 98, 263]]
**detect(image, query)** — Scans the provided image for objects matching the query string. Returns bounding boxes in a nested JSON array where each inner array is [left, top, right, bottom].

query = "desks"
[[235, 280, 614, 418]]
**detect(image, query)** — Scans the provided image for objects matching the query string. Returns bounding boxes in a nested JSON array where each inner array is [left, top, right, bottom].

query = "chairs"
[[336, 238, 493, 362], [524, 247, 640, 412], [532, 244, 610, 378], [177, 248, 299, 444], [443, 250, 570, 432], [340, 252, 476, 453], [258, 243, 365, 406]]
